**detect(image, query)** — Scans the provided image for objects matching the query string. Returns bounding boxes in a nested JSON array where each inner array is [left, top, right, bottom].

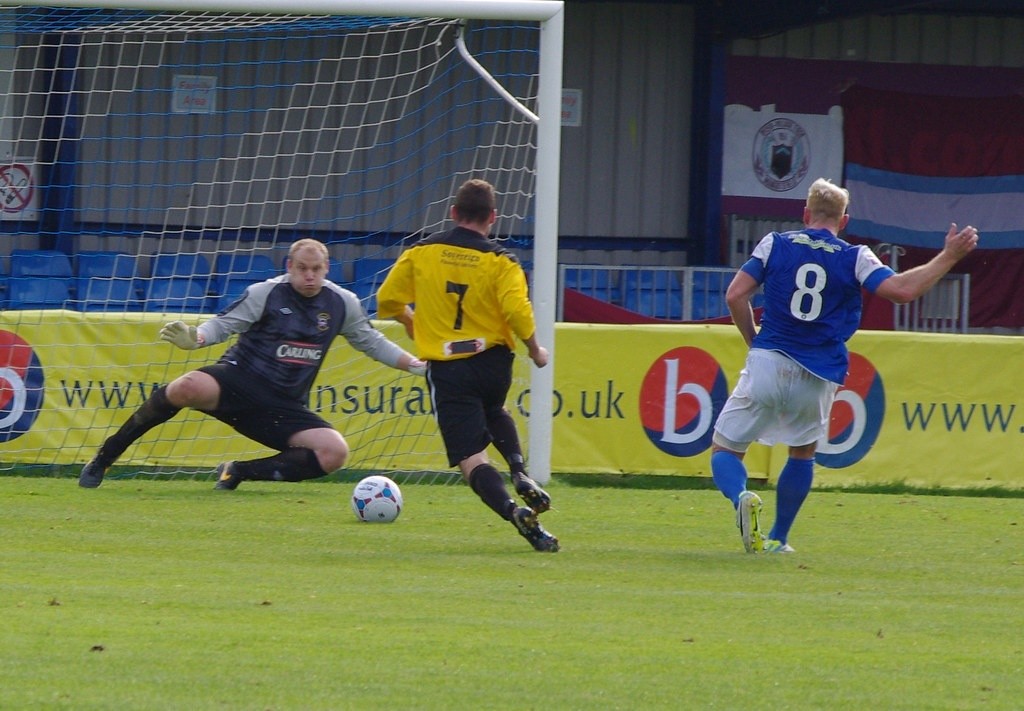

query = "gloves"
[[156, 321, 200, 353]]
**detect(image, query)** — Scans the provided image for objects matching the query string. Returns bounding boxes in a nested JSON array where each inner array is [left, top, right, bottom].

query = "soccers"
[[351, 475, 403, 523]]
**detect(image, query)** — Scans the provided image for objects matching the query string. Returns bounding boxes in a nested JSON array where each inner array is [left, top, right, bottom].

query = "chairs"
[[2, 248, 761, 326]]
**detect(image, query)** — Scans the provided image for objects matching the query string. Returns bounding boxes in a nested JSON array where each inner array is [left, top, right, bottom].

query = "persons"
[[373, 177, 563, 555], [75, 237, 431, 492], [709, 173, 979, 558]]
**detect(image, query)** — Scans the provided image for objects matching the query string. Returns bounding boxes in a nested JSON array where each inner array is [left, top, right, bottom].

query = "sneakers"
[[212, 461, 244, 489], [513, 472, 551, 513], [762, 540, 796, 553], [512, 506, 561, 553], [735, 490, 767, 553], [79, 441, 115, 487]]
